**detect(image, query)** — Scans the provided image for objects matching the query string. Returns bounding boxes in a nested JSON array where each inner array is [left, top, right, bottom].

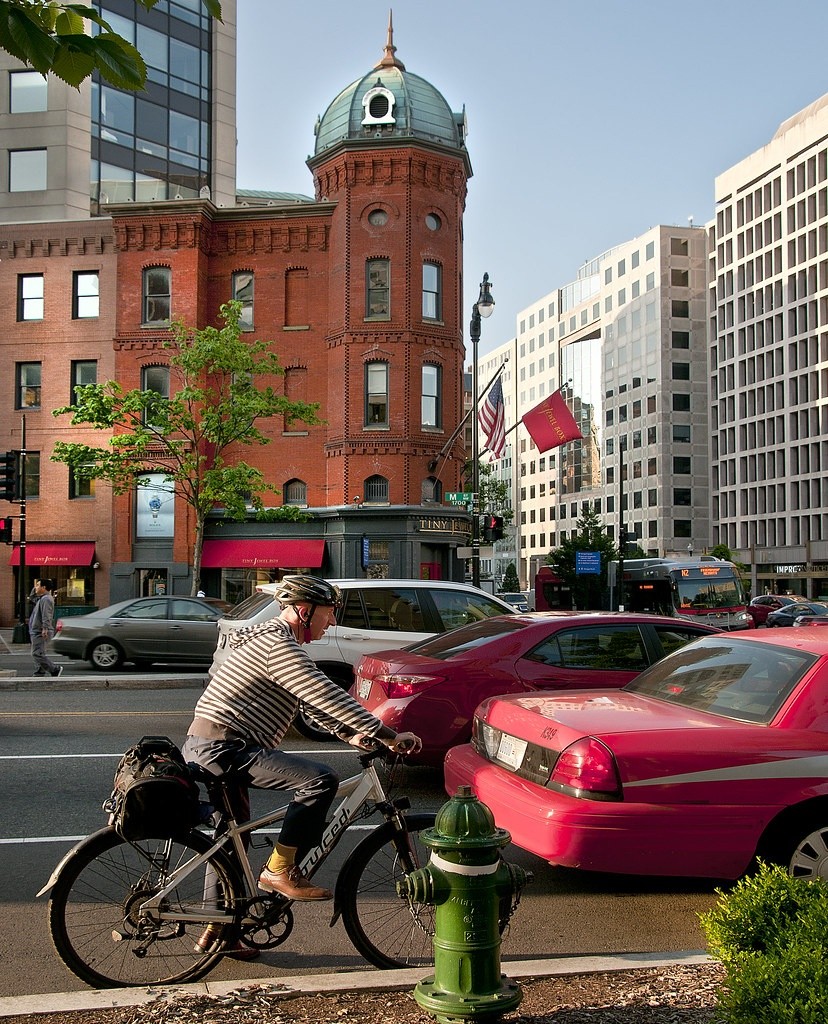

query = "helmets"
[[274, 574, 344, 608]]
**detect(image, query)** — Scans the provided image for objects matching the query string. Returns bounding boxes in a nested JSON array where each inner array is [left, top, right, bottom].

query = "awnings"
[[200, 539, 325, 568], [7, 542, 96, 566]]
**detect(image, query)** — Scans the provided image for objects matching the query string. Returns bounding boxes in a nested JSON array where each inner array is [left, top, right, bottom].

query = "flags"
[[521, 389, 585, 455], [478, 375, 506, 462]]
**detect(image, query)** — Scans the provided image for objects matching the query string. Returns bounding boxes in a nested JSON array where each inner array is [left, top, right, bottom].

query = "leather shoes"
[[193, 931, 260, 961], [257, 864, 333, 901]]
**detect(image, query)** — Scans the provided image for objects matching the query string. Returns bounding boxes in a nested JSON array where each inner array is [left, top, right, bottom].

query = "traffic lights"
[[627, 531, 638, 555], [485, 516, 504, 541], [0, 452, 16, 499], [0, 518, 8, 542]]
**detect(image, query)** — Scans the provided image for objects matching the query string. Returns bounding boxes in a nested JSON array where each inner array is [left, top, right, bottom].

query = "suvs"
[[202, 578, 523, 742], [747, 594, 812, 628]]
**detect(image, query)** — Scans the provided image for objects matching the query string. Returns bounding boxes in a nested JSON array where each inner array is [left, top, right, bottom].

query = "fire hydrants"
[[394, 785, 535, 1024]]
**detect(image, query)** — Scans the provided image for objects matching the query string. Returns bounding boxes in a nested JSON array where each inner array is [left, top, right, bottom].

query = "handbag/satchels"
[[113, 735, 201, 842]]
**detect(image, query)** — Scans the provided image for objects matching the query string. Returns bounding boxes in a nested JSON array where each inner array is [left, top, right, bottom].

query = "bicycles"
[[36, 736, 514, 991]]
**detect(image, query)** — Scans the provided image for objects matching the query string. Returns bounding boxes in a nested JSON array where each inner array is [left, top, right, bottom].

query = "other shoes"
[[33, 669, 45, 676], [52, 666, 63, 677]]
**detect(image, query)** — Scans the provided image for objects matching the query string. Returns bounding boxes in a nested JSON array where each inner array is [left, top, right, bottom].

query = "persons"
[[180, 575, 422, 962], [29, 579, 64, 677]]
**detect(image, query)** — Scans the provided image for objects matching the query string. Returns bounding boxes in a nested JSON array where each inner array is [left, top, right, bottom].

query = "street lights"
[[686, 542, 694, 556], [470, 271, 497, 587]]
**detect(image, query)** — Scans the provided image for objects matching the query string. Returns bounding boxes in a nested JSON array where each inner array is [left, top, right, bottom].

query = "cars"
[[494, 592, 530, 613], [49, 595, 226, 673], [793, 615, 828, 627], [765, 601, 828, 627], [443, 626, 828, 902], [343, 608, 728, 786]]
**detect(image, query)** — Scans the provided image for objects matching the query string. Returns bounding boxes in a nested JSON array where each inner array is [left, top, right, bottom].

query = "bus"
[[535, 557, 756, 634]]
